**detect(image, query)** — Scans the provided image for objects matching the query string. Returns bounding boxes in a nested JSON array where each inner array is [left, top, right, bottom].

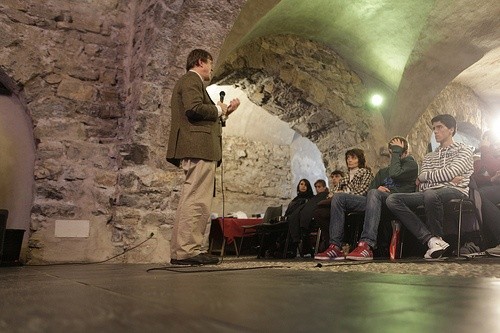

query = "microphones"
[[220, 91, 225, 103]]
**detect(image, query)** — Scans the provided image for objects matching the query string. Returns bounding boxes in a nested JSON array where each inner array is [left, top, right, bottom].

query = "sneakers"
[[451, 242, 487, 256], [485, 244, 500, 256], [177, 251, 219, 265], [314, 242, 346, 260], [346, 240, 374, 260]]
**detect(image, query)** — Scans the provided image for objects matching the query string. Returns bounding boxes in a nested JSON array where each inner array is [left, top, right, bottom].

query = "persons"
[[284, 114, 500, 261], [166, 49, 240, 265]]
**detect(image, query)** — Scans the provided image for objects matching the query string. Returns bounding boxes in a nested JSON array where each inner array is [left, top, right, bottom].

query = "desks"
[[209, 218, 264, 256]]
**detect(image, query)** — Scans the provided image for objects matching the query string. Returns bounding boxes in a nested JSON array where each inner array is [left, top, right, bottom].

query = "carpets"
[[204, 253, 500, 279]]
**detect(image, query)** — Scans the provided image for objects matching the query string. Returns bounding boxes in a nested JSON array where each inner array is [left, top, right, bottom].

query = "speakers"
[[0, 229, 26, 266]]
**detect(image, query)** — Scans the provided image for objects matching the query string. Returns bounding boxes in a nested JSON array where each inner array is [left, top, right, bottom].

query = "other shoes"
[[424, 235, 442, 259], [428, 237, 450, 258], [170, 257, 177, 264]]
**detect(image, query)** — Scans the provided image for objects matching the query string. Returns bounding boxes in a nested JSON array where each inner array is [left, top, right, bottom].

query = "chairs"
[[238, 185, 500, 260]]
